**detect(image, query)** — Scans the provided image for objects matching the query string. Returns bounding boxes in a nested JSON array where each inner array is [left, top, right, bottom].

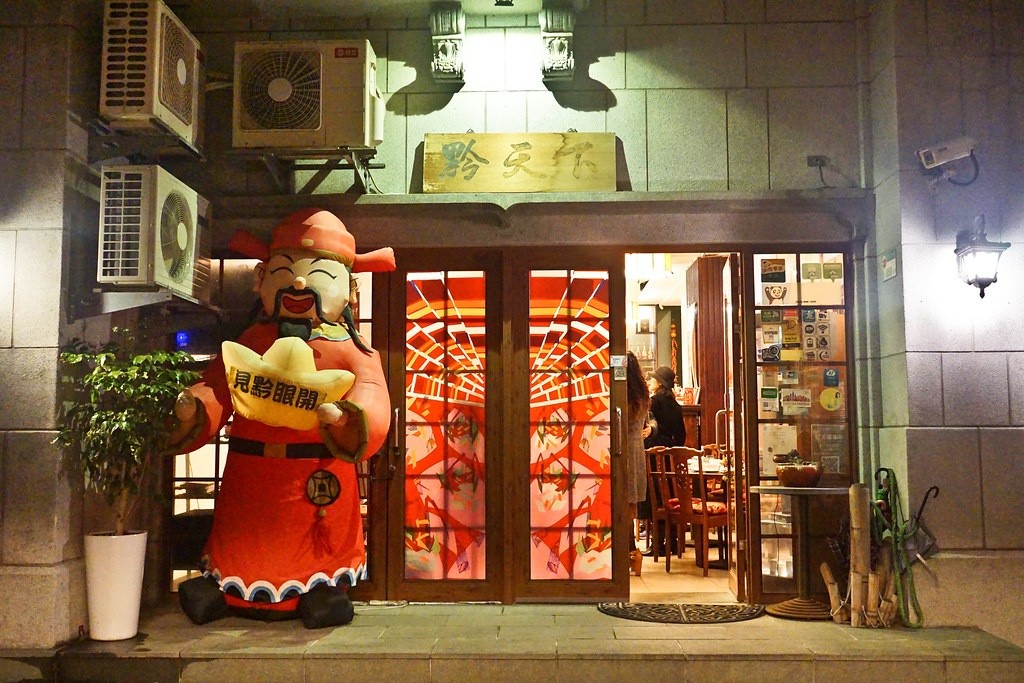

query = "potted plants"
[[50, 324, 204, 642]]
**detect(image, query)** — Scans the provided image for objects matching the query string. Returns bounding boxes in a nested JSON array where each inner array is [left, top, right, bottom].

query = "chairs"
[[645, 443, 730, 577]]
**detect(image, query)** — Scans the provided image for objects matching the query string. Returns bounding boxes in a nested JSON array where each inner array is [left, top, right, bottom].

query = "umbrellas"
[[872, 468, 940, 603]]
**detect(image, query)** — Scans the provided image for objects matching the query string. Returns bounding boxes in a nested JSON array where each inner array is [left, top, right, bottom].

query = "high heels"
[[629, 548, 642, 576]]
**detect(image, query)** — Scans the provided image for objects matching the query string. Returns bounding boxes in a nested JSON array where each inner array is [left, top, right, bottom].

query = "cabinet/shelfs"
[[629, 257, 728, 446]]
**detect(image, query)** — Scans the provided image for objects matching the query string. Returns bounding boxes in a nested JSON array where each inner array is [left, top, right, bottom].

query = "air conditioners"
[[99, 0, 206, 157], [96, 165, 212, 302], [233, 39, 385, 149]]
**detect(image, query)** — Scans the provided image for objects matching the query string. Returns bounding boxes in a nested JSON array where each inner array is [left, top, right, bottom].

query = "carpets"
[[594, 603, 766, 625]]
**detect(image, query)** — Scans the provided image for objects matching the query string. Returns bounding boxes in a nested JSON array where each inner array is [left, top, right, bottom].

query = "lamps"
[[954, 216, 1012, 298], [495, 0, 514, 7]]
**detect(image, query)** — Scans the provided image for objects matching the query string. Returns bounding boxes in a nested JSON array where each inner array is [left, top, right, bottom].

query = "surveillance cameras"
[[918, 137, 976, 169]]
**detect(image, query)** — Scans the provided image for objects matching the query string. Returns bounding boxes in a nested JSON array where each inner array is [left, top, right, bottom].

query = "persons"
[[624, 351, 688, 578], [168, 209, 397, 626]]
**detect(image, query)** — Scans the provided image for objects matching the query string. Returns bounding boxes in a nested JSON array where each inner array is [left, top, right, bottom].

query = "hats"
[[648, 366, 674, 389]]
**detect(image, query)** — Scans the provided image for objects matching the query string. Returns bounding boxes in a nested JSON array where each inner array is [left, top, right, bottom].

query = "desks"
[[749, 485, 850, 620], [650, 472, 728, 570]]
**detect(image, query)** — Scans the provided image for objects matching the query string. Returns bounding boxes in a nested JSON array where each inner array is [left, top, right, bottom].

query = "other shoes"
[[642, 538, 665, 556], [664, 540, 678, 555]]
